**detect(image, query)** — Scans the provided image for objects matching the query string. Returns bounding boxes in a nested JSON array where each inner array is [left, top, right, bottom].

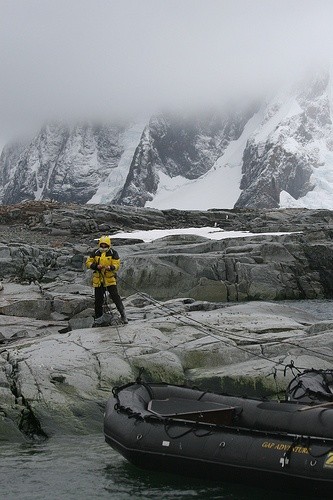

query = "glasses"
[[100, 244, 108, 248]]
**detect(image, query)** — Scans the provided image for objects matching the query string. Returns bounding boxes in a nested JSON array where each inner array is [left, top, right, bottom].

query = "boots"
[[92, 315, 97, 328], [118, 309, 128, 324]]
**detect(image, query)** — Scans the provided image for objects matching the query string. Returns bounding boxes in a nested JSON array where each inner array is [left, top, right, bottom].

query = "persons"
[[85, 235, 129, 327]]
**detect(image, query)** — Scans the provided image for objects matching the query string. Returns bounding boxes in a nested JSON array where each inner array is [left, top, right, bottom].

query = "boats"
[[101, 380, 333, 486]]
